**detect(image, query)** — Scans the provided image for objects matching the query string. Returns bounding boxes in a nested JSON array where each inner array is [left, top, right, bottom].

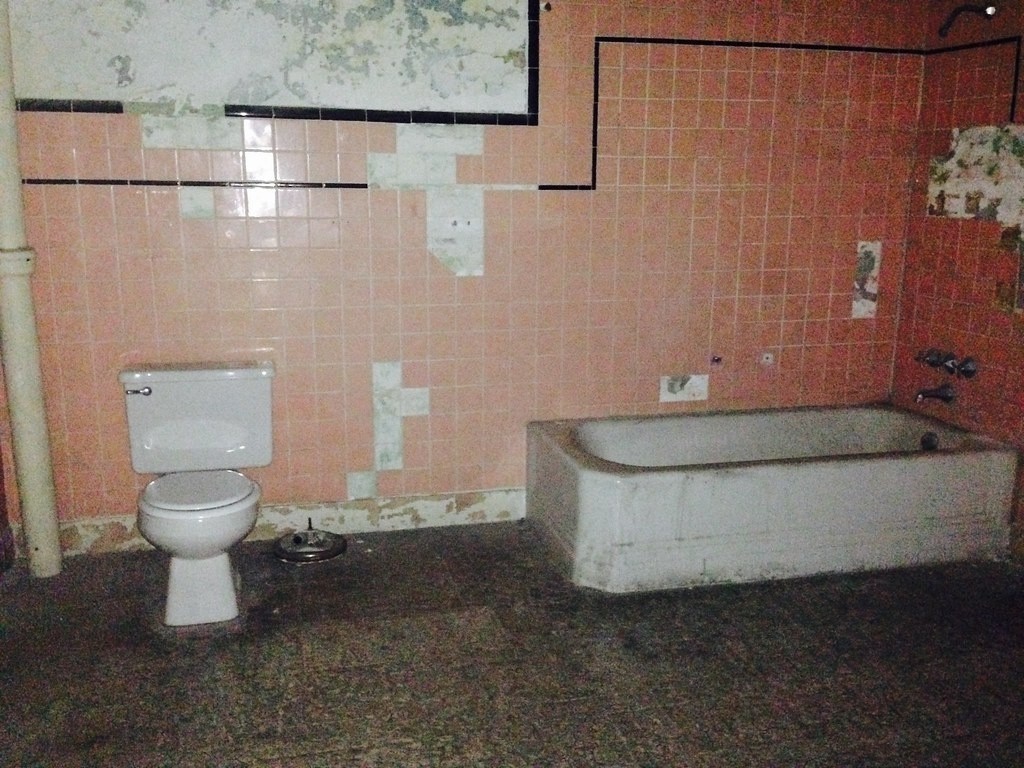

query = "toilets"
[[118, 357, 275, 626]]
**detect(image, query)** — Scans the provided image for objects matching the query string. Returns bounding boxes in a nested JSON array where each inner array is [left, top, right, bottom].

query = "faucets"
[[916, 384, 953, 403]]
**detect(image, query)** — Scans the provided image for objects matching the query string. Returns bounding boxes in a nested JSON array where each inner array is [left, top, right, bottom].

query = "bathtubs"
[[527, 405, 1017, 596]]
